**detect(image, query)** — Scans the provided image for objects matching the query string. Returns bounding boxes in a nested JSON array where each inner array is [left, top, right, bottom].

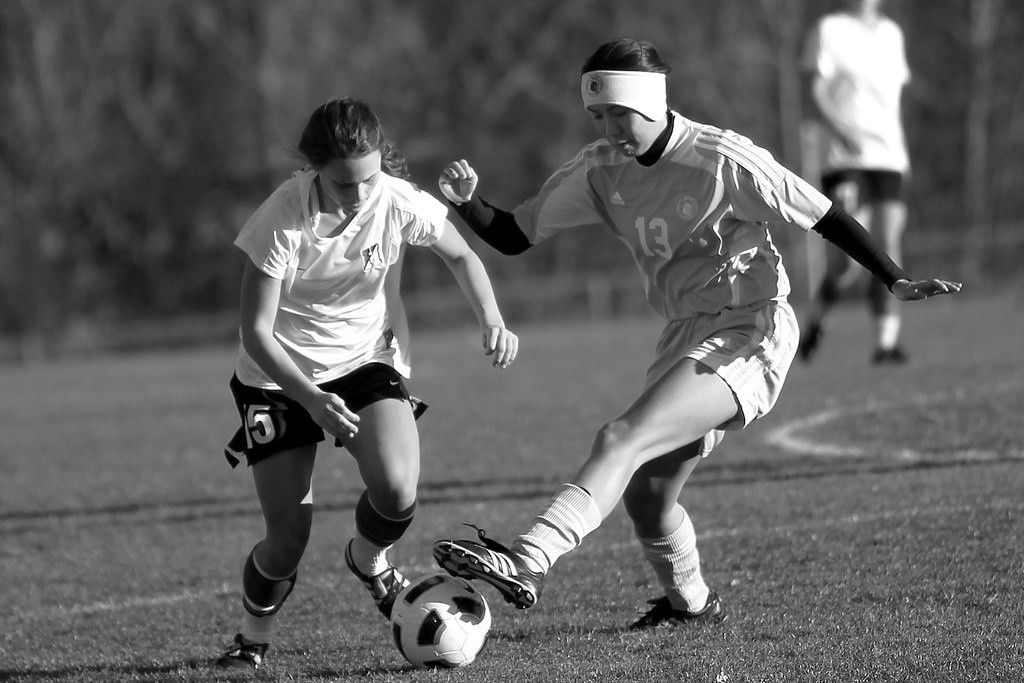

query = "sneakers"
[[628, 589, 728, 631], [432, 523, 545, 610], [344, 537, 412, 622], [215, 632, 272, 671]]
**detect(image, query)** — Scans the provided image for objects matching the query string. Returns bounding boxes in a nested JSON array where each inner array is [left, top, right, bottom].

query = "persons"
[[799, 0, 913, 364], [433, 37, 962, 631], [217, 99, 519, 671]]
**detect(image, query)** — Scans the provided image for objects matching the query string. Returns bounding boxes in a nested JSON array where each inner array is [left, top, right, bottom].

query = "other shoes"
[[872, 346, 907, 365], [799, 323, 822, 357]]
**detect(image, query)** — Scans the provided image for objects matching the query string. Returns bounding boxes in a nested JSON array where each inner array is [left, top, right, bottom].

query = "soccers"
[[389, 571, 493, 670]]
